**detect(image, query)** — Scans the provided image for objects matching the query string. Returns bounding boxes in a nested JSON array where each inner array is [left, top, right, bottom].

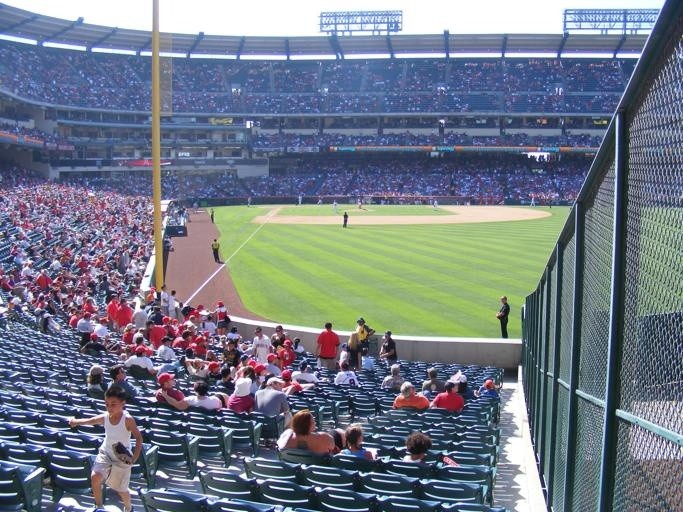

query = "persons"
[[2, 7, 682, 417], [334, 426, 373, 461], [276, 408, 347, 454], [68, 385, 143, 512], [403, 433, 431, 461]]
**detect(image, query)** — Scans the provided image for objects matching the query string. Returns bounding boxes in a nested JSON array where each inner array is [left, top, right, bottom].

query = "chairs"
[[1, 168, 157, 325]]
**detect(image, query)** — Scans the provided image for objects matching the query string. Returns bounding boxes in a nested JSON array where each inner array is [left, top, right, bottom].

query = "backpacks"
[[181, 306, 195, 316]]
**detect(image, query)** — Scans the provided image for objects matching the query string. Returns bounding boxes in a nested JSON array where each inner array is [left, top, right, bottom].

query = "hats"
[[283, 340, 291, 346], [84, 312, 91, 317], [356, 318, 365, 323], [126, 323, 136, 329], [485, 380, 494, 388], [90, 333, 97, 339], [162, 316, 171, 323], [267, 376, 284, 386], [254, 365, 266, 373], [198, 305, 204, 309], [217, 301, 223, 306], [161, 336, 173, 342], [101, 318, 110, 323], [158, 373, 175, 384], [281, 370, 291, 379], [135, 346, 148, 352], [267, 354, 277, 361], [89, 365, 103, 375], [427, 367, 436, 374], [208, 362, 219, 371], [298, 360, 307, 371], [234, 378, 252, 397]]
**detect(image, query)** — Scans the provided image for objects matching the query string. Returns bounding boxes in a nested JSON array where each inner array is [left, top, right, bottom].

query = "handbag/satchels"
[[224, 316, 230, 323]]
